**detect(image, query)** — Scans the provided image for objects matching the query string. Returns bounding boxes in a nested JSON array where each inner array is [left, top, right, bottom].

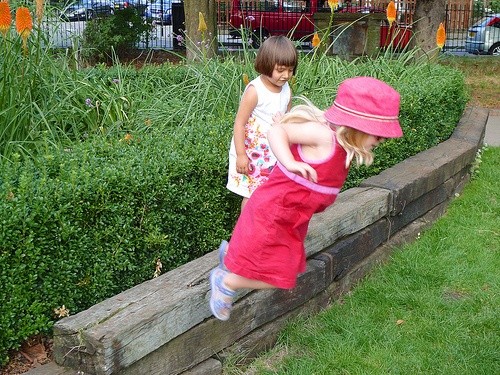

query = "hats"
[[323, 76, 403, 138]]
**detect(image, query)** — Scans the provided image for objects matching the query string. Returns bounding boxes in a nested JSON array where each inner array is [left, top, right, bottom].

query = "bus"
[[229, 0, 415, 51]]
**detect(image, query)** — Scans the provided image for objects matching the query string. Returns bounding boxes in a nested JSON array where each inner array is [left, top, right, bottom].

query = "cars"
[[65, 0, 114, 21], [145, 0, 181, 25], [114, 0, 149, 17], [465, 13, 500, 54]]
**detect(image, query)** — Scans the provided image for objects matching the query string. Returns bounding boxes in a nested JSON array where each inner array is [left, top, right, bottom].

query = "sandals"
[[210, 267, 236, 321], [219, 239, 230, 272]]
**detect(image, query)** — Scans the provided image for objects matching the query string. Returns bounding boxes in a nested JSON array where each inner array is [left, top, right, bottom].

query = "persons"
[[209, 77, 403, 322], [226, 36, 298, 214]]
